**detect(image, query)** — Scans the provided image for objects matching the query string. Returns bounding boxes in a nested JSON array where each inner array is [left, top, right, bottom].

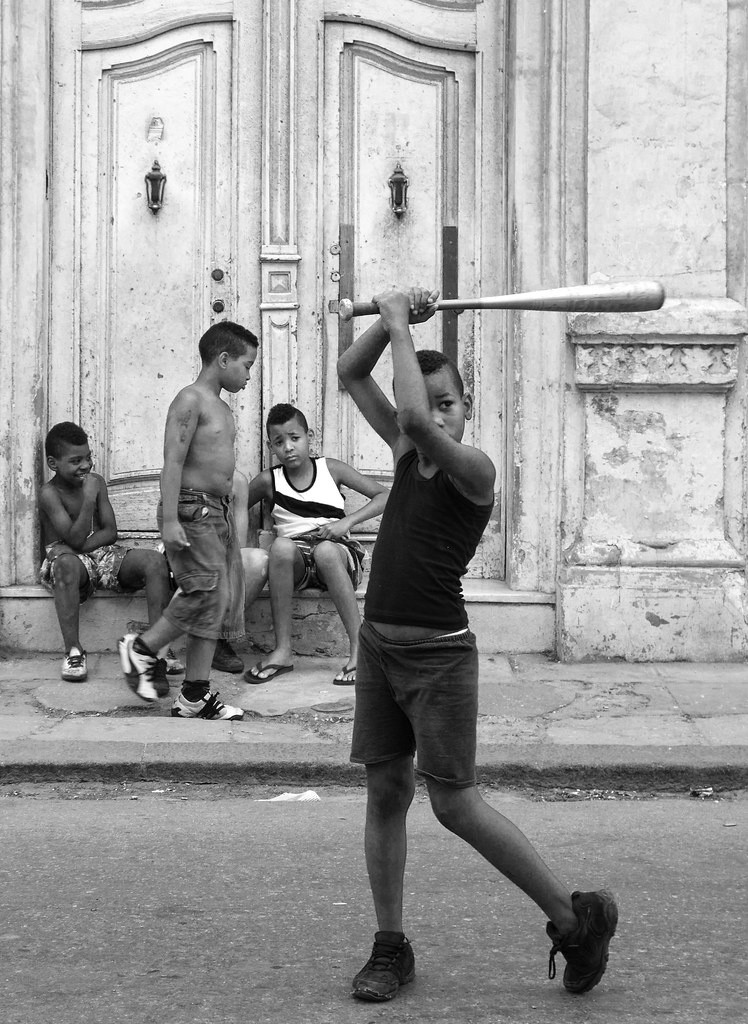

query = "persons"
[[115, 322, 260, 720], [211, 470, 269, 672], [244, 404, 390, 686], [336, 285, 620, 1000], [37, 421, 184, 680]]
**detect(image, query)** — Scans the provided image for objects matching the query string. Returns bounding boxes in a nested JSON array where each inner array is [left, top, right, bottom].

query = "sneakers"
[[545, 887, 619, 993], [165, 648, 185, 674], [211, 639, 245, 673], [350, 930, 416, 1002], [116, 632, 159, 702], [149, 655, 170, 697], [169, 690, 244, 721], [61, 649, 88, 681]]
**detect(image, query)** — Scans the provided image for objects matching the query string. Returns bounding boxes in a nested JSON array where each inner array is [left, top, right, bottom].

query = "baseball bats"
[[337, 278, 668, 323]]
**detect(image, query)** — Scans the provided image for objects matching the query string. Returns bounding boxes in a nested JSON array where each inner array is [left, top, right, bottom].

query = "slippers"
[[332, 666, 357, 685], [243, 661, 294, 684]]
